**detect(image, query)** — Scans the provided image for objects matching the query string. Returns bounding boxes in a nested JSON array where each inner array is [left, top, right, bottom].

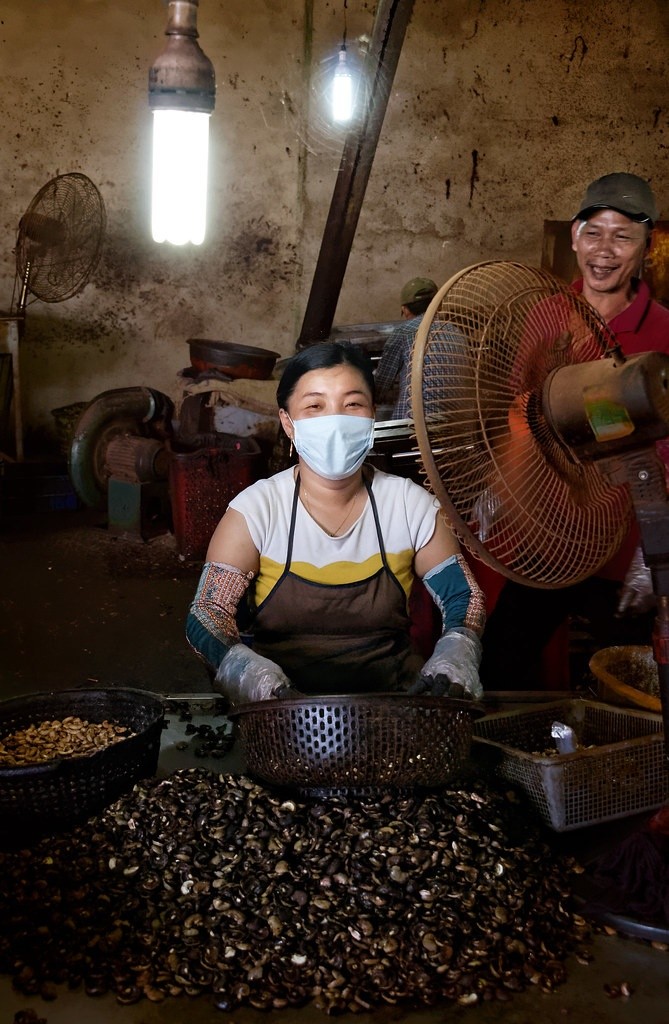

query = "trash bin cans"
[[164, 433, 262, 561]]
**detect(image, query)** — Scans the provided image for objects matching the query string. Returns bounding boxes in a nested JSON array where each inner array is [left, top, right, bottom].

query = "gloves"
[[618, 547, 653, 613], [407, 626, 485, 701], [475, 490, 514, 541], [214, 643, 292, 702]]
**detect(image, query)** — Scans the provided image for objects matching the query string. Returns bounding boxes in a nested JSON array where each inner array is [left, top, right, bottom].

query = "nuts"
[[0, 714, 138, 768]]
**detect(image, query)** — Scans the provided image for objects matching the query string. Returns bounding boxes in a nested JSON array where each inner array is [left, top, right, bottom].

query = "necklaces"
[[299, 467, 362, 538]]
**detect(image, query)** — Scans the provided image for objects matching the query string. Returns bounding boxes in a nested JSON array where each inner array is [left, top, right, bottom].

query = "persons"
[[375, 277, 470, 420], [469, 172, 668, 702], [186, 342, 487, 702]]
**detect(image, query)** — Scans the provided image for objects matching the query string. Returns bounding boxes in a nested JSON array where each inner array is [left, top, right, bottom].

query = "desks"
[[0, 317, 24, 461]]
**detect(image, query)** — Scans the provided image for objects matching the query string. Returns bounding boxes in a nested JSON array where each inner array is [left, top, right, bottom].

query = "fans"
[[0, 173, 107, 449], [403, 258, 669, 944]]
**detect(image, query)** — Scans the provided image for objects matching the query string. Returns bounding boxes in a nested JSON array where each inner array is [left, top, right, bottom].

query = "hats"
[[571, 172, 656, 228]]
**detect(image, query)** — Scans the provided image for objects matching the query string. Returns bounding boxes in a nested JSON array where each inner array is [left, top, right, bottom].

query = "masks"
[[285, 410, 375, 481]]
